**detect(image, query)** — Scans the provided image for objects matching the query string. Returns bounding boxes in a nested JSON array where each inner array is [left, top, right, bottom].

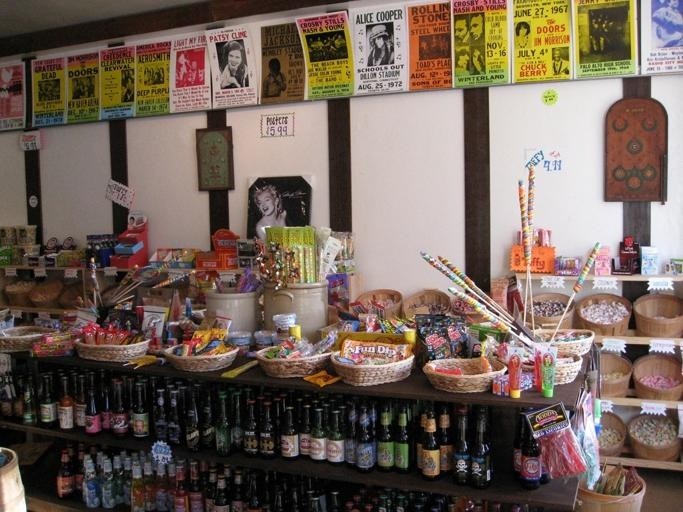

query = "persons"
[[651, 0, 683, 47], [552, 49, 568, 75], [252, 185, 286, 240], [453, 15, 485, 74], [366, 25, 393, 66], [590, 14, 613, 55], [122, 76, 134, 101], [220, 41, 247, 89], [515, 22, 530, 48], [262, 58, 286, 97], [307, 33, 347, 62]]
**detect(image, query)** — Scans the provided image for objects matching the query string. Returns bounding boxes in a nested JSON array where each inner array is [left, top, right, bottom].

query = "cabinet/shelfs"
[[0, 337, 594, 512], [506, 259, 681, 477], [0, 256, 267, 336]]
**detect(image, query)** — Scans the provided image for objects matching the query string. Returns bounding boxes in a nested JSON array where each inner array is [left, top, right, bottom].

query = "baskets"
[[164, 344, 240, 373], [354, 289, 403, 322], [422, 357, 508, 393], [58, 283, 83, 308], [0, 326, 60, 353], [256, 346, 335, 379], [633, 355, 683, 400], [452, 294, 491, 324], [330, 350, 415, 387], [504, 350, 584, 386], [74, 337, 151, 363], [3, 280, 37, 306], [28, 279, 63, 308], [0, 275, 17, 305], [633, 294, 683, 338], [522, 293, 575, 329], [575, 293, 632, 336], [401, 289, 450, 323], [524, 321, 541, 332], [627, 414, 683, 461], [532, 329, 594, 356], [598, 411, 626, 457], [600, 352, 633, 398]]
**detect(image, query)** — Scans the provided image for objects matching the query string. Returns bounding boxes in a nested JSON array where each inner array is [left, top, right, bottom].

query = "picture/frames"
[[245, 173, 314, 240], [194, 125, 236, 191]]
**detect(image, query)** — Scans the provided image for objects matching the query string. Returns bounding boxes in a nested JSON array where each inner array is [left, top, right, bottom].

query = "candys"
[[90, 250, 196, 293], [420, 165, 601, 332]]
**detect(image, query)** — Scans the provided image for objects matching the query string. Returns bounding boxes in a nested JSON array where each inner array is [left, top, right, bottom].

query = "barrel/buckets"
[[263, 280, 330, 344], [204, 290, 258, 348]]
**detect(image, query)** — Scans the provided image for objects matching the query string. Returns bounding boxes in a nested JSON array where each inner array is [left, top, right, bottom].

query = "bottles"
[[53, 440, 544, 512], [1, 362, 548, 490]]
